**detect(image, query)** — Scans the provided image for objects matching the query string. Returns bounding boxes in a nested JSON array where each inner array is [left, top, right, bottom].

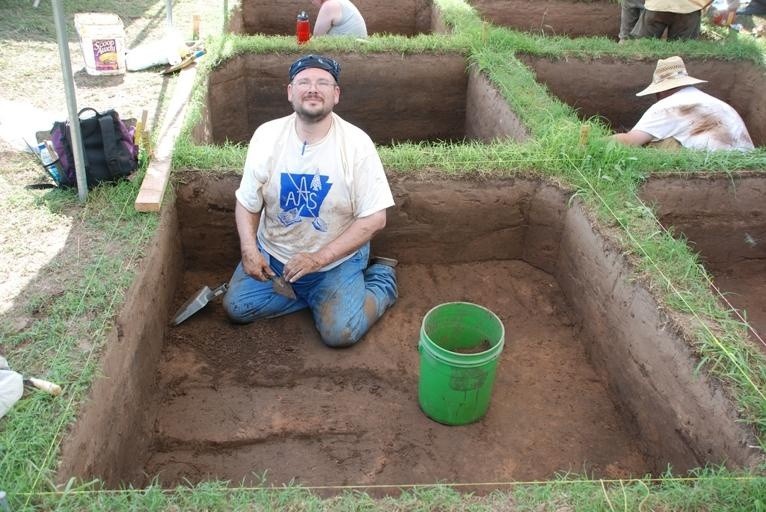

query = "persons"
[[605, 56, 755, 151], [618, 0, 714, 42], [311, 0, 368, 36], [222, 54, 400, 350]]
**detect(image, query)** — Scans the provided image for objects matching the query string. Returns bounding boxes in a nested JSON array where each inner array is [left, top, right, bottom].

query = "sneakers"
[[370, 256, 398, 267]]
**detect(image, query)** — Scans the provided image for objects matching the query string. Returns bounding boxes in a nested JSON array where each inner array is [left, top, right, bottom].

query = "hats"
[[635, 56, 709, 97]]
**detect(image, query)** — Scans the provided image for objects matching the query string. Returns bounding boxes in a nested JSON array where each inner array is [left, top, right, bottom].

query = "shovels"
[[169, 282, 229, 328], [160, 48, 205, 77], [23, 376, 63, 395]]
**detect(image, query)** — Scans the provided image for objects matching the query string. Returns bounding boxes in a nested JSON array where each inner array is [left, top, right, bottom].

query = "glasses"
[[291, 79, 336, 88]]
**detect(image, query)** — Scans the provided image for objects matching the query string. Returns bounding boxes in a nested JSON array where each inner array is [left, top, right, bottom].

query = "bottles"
[[38, 142, 60, 182], [297, 11, 311, 42]]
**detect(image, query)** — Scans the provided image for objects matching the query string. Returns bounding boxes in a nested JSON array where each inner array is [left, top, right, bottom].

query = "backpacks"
[[50, 107, 138, 186]]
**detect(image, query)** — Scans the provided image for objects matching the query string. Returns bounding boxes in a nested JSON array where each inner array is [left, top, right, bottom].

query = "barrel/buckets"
[[73, 12, 126, 75], [418, 300, 507, 426]]
